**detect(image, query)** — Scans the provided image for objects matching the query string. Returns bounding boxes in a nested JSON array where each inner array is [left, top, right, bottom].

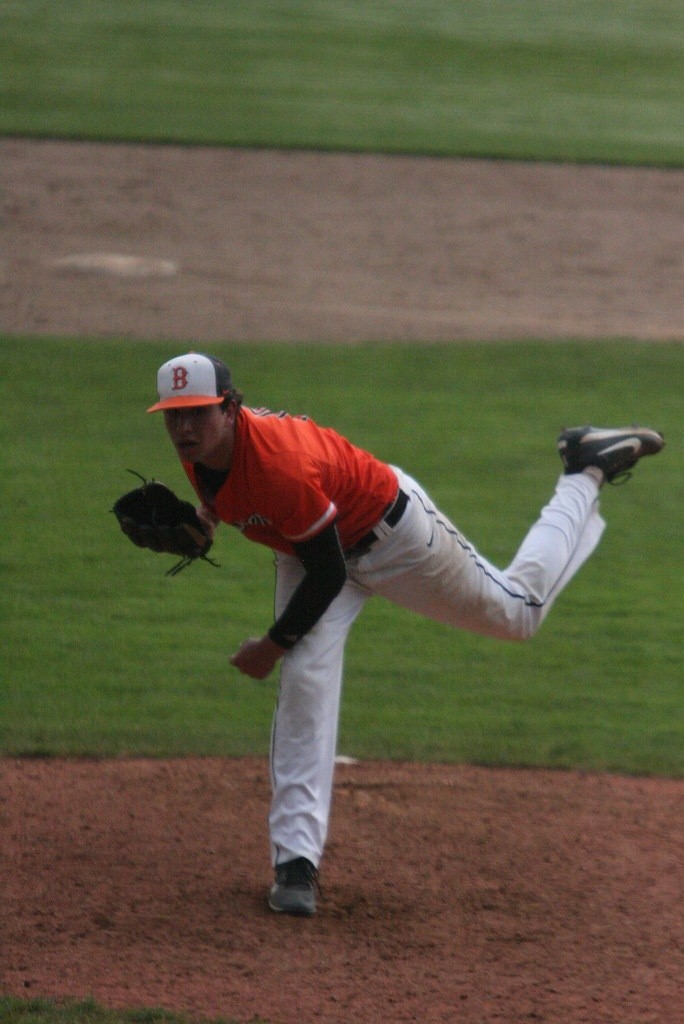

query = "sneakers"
[[268, 857, 324, 916], [556, 426, 665, 486]]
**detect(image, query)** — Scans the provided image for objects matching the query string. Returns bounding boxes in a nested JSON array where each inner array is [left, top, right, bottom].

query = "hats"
[[148, 350, 234, 414]]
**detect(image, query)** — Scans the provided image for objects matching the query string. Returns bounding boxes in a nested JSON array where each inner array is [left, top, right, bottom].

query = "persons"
[[146, 351, 667, 915]]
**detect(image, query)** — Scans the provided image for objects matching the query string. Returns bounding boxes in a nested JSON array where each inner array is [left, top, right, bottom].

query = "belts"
[[342, 489, 410, 556]]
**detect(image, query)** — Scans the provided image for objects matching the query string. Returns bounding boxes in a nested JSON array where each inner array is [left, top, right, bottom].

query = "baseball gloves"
[[112, 482, 214, 559]]
